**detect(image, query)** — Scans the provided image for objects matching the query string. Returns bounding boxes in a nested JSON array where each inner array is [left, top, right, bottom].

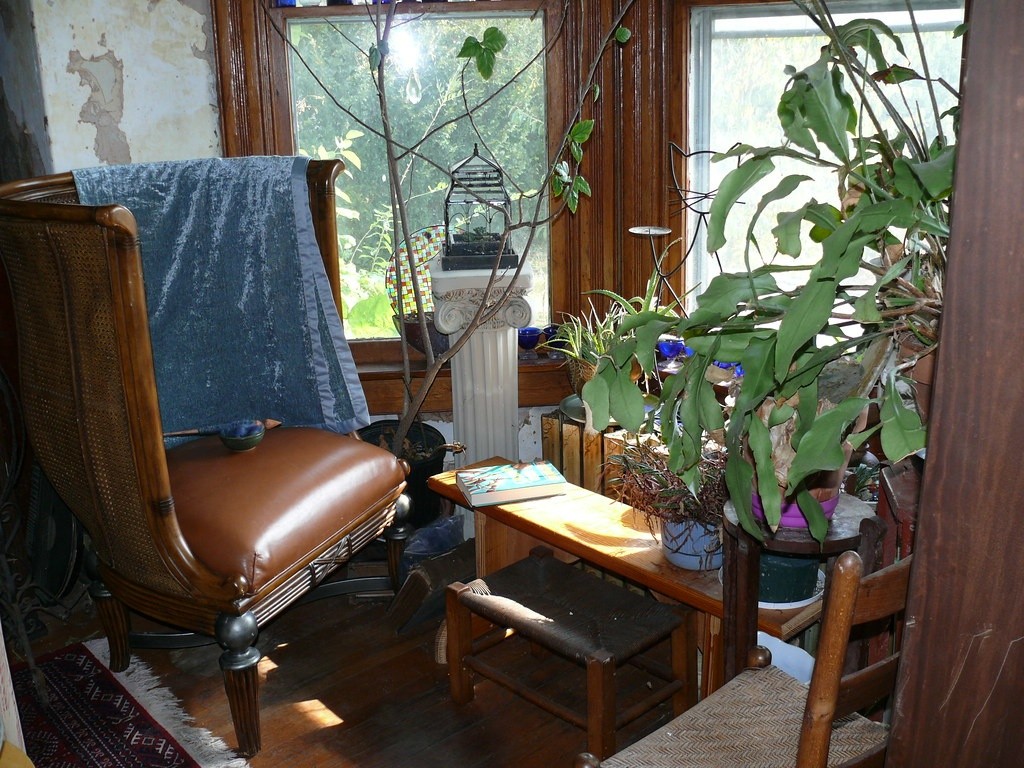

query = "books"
[[456, 459, 568, 508]]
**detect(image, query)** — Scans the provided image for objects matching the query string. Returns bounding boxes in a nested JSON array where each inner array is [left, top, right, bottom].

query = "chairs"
[[0, 155, 412, 759], [576, 551, 913, 768]]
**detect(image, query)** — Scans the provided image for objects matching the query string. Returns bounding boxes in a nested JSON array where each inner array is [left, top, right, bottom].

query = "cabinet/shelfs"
[[448, 546, 693, 761]]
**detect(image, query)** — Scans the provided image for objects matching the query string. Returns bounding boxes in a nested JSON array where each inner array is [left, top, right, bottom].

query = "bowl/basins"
[[392, 313, 449, 366], [219, 429, 265, 454]]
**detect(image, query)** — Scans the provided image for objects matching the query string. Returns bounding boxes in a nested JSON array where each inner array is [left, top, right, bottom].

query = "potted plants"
[[534, 0, 968, 574], [256, 0, 638, 523]]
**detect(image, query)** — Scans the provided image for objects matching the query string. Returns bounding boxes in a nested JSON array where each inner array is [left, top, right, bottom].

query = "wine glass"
[[518, 327, 543, 360], [658, 341, 683, 369], [543, 326, 565, 360]]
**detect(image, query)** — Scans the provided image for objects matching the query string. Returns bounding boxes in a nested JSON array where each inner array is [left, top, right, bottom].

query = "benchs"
[[428, 453, 834, 704]]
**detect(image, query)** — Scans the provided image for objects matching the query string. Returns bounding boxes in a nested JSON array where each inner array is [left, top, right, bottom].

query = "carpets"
[[10, 635, 255, 768]]
